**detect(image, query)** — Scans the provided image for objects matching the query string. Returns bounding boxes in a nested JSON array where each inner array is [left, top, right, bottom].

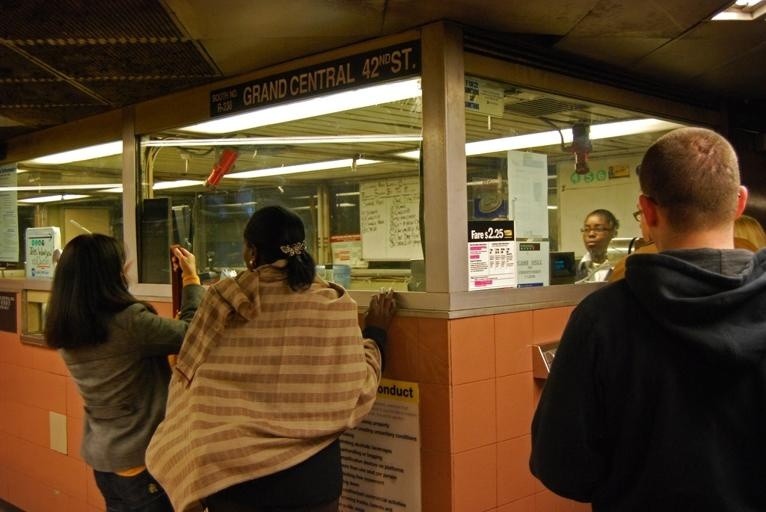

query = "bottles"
[[325, 264, 333, 281]]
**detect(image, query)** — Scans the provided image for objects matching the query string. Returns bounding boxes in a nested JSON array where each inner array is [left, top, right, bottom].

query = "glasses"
[[632, 196, 659, 223], [581, 226, 612, 232]]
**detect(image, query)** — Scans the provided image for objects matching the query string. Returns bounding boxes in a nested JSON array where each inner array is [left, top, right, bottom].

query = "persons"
[[530, 126, 765, 511], [46, 234, 205, 512], [146, 206, 397, 512]]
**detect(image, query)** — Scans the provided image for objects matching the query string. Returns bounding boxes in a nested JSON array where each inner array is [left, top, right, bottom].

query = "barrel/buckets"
[[333, 265, 350, 289]]
